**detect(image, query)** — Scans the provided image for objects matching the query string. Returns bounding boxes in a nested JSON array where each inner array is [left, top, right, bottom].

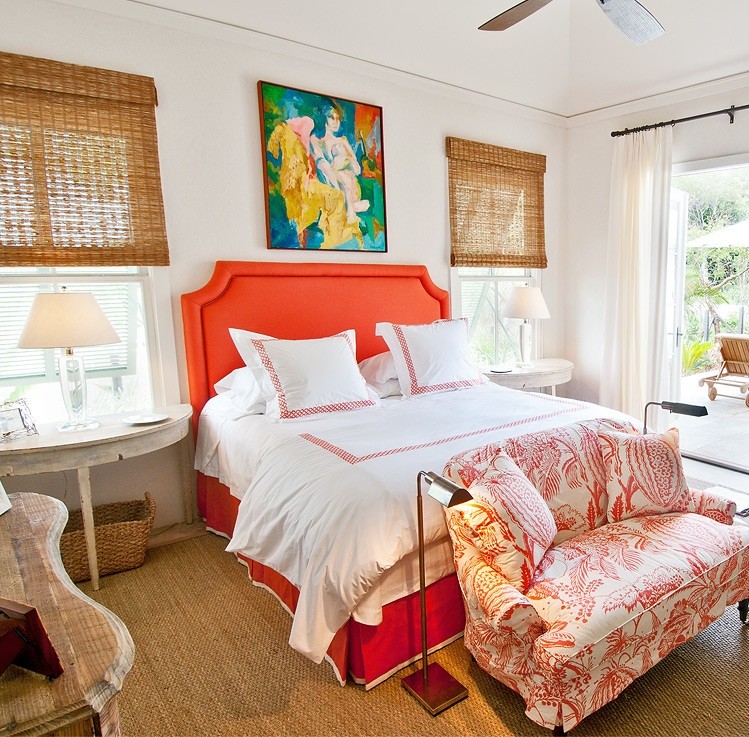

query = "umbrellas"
[[686, 219, 749, 334]]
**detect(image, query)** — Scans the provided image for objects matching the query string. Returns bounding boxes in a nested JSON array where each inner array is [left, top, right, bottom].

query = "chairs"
[[698, 333, 749, 408]]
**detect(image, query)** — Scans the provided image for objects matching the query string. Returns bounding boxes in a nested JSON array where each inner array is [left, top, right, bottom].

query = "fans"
[[477, 0, 666, 46]]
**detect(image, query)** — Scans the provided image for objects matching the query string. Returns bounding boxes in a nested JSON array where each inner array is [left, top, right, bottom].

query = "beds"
[[180, 260, 652, 692]]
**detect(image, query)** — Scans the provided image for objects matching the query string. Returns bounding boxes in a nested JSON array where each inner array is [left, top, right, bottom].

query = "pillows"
[[212, 318, 490, 423], [449, 448, 558, 594], [597, 428, 697, 523]]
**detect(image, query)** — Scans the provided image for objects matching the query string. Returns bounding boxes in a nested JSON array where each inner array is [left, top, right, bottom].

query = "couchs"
[[441, 418, 749, 737]]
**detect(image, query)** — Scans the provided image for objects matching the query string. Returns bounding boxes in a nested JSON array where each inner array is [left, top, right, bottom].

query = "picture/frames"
[[0, 598, 65, 679], [0, 398, 38, 445], [257, 80, 388, 253]]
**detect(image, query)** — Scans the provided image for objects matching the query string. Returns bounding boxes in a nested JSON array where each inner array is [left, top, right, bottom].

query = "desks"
[[486, 359, 574, 396], [1, 402, 194, 591], [0, 491, 135, 737]]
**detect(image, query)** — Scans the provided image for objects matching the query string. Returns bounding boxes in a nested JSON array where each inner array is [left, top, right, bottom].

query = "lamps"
[[400, 470, 474, 716], [501, 287, 553, 367], [644, 401, 709, 436], [16, 286, 122, 432]]
